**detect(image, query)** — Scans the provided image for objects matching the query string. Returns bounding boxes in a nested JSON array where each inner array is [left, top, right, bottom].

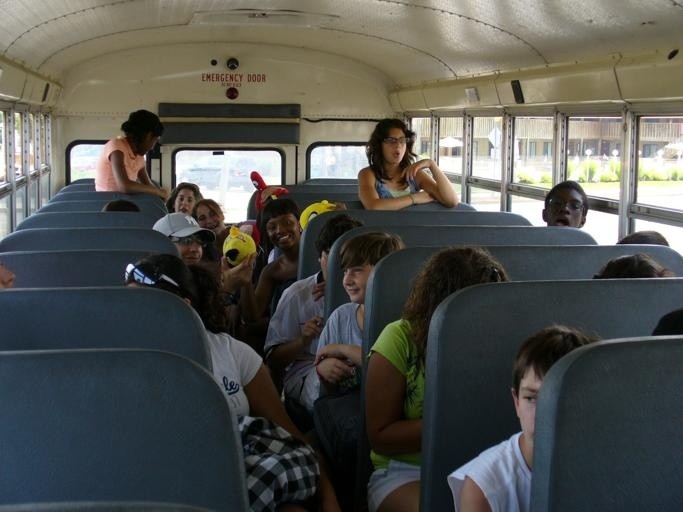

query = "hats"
[[151, 212, 216, 247]]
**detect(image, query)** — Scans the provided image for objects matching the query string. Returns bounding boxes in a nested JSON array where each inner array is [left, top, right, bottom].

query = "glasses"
[[123, 261, 180, 287], [381, 136, 410, 146], [172, 235, 208, 249]]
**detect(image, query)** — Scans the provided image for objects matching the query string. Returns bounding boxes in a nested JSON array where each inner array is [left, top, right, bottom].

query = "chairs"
[[3, 283, 215, 374], [0, 347, 242, 508], [250, 182, 683, 511], [1, 246, 184, 289], [1, 177, 178, 247]]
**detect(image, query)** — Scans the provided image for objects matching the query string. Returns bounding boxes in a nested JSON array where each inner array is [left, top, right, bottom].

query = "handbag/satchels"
[[314, 379, 359, 464]]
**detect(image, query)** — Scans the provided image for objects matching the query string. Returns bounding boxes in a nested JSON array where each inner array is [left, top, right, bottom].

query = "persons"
[[313, 230, 411, 394], [95, 110, 168, 201], [651, 308, 683, 336], [263, 215, 367, 423], [0, 262, 16, 289], [124, 252, 342, 512], [359, 116, 459, 211], [616, 230, 670, 247], [542, 183, 588, 228], [590, 252, 676, 279], [150, 183, 347, 358], [363, 246, 507, 512], [445, 323, 600, 512]]
